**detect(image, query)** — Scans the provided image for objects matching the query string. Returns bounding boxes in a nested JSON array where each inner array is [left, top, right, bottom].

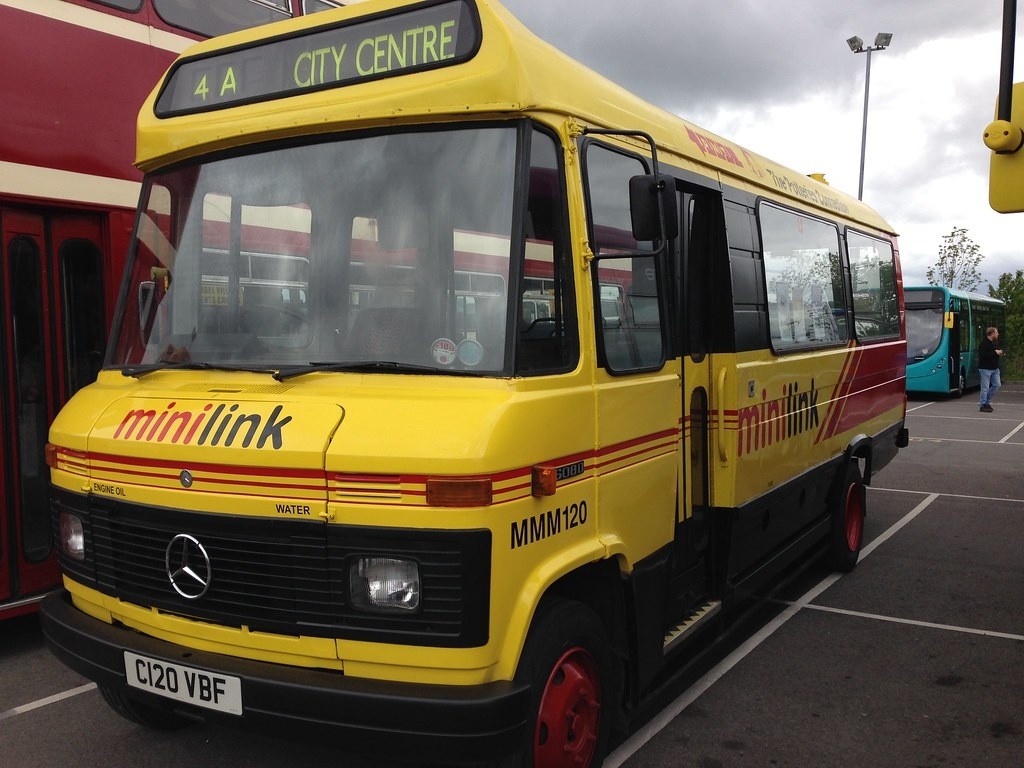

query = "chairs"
[[343, 307, 431, 366], [520, 317, 570, 371]]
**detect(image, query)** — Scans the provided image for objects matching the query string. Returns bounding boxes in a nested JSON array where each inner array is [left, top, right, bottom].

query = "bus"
[[1, 0, 635, 619], [40, 0, 909, 768], [838, 286, 1007, 400], [828, 305, 897, 338]]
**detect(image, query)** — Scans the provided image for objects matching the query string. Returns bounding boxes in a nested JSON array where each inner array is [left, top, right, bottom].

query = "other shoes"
[[980, 405, 993, 412]]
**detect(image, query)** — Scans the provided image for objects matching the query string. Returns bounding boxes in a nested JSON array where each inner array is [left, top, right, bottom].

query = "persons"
[[978, 328, 1006, 412]]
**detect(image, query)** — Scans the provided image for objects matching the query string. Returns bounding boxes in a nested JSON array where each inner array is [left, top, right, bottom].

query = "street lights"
[[844, 32, 894, 201]]
